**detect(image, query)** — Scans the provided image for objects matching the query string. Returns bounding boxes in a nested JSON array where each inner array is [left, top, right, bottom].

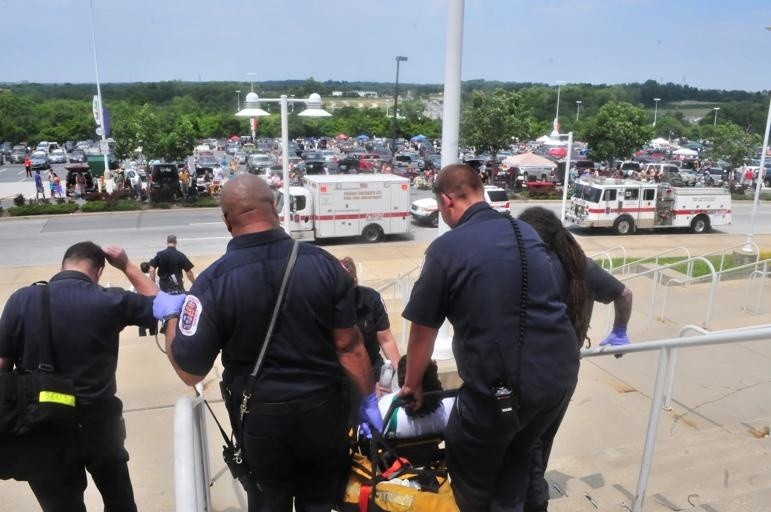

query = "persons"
[[150, 172, 376, 512], [0, 239, 161, 512], [400, 163, 580, 512], [138, 261, 155, 336], [150, 234, 195, 291], [516, 206, 633, 511], [339, 256, 401, 389], [362, 352, 446, 462]]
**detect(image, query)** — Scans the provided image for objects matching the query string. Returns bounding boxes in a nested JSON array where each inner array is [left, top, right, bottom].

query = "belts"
[[246, 387, 333, 417]]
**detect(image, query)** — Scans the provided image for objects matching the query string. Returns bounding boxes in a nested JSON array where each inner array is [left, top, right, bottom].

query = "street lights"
[[712, 106, 723, 127], [290, 93, 297, 112], [247, 73, 260, 92], [236, 90, 242, 113], [554, 79, 567, 130], [557, 131, 574, 228], [576, 100, 584, 121], [744, 27, 771, 252], [391, 55, 409, 156], [653, 97, 662, 128]]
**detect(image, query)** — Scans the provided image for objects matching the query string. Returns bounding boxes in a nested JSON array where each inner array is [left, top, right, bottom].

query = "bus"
[[565, 167, 734, 235]]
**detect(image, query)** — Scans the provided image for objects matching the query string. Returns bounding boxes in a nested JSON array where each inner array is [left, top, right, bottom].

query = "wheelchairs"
[[358, 386, 463, 485]]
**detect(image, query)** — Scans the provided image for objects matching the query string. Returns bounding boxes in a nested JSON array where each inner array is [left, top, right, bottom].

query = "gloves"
[[599, 325, 630, 359], [356, 392, 386, 438], [152, 290, 187, 322]]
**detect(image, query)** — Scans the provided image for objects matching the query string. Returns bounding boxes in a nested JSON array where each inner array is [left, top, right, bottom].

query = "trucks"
[[276, 174, 411, 243]]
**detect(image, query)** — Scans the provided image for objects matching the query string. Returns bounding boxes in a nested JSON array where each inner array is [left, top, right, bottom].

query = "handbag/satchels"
[[0, 281, 81, 444], [331, 421, 461, 512]]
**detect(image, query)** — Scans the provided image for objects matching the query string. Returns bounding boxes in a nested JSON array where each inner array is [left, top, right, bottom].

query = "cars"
[[193, 135, 442, 174], [136, 160, 162, 179], [0, 139, 92, 171], [136, 140, 144, 149]]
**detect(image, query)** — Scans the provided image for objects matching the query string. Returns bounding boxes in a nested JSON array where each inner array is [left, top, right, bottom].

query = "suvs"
[[64, 165, 97, 195], [411, 184, 511, 230], [149, 163, 181, 190], [99, 169, 143, 196]]
